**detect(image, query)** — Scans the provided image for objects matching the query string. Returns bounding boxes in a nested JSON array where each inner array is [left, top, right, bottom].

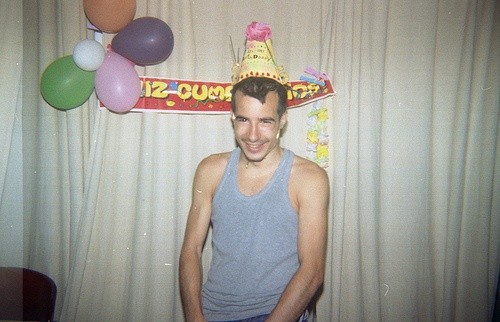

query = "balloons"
[[111, 16, 173, 67], [41, 55, 95, 112], [73, 39, 105, 72], [95, 48, 143, 114], [82, 0, 137, 34]]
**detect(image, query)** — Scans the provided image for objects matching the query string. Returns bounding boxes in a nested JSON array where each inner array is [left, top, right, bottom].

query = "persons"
[[178, 77, 330, 322]]
